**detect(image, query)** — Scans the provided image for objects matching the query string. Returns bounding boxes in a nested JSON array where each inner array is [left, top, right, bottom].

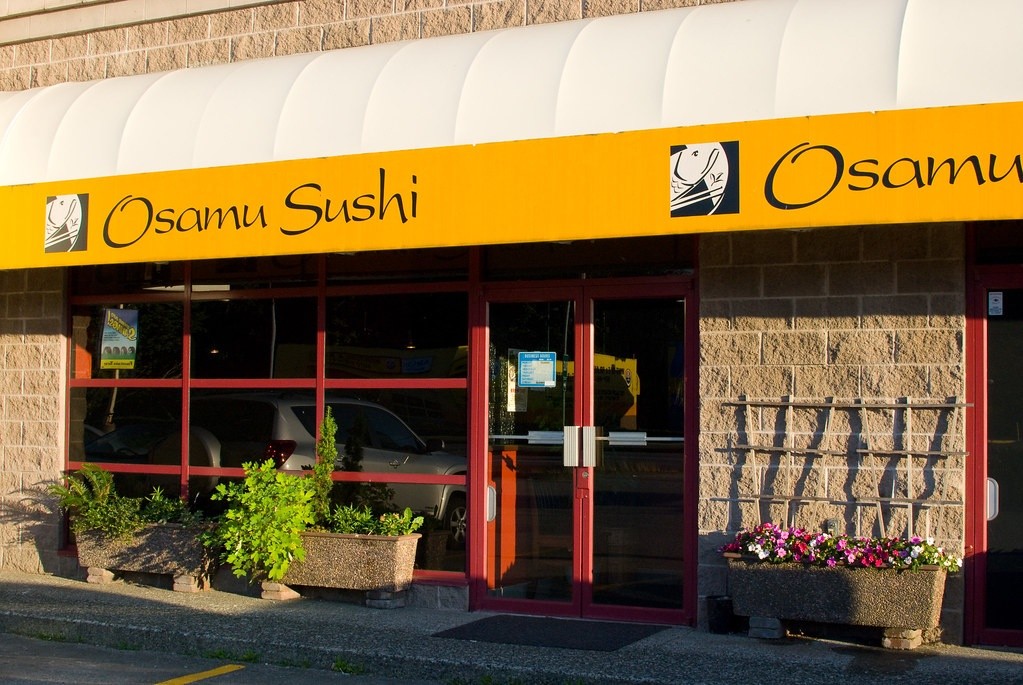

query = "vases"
[[724, 552, 948, 630]]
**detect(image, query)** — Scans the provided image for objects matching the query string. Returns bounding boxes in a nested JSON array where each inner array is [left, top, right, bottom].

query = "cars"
[[84, 423, 146, 499]]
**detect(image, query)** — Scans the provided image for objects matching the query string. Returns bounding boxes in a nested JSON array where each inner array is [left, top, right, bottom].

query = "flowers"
[[717, 522, 964, 571]]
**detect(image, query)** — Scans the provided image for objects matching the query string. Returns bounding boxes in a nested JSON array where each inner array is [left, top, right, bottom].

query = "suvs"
[[146, 394, 468, 550]]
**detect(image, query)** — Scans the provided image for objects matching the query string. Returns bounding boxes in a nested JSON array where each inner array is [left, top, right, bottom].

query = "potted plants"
[[46, 461, 221, 578], [211, 406, 423, 591]]
